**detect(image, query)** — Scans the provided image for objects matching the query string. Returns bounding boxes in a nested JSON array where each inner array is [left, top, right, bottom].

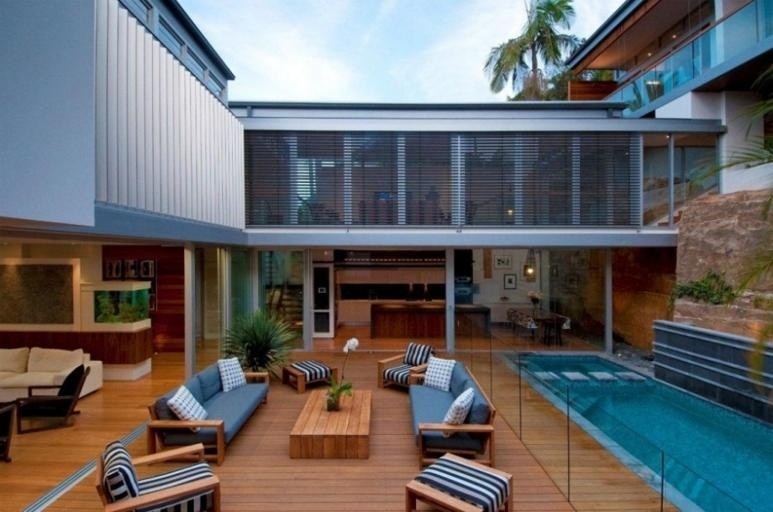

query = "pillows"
[[450, 363, 469, 399], [197, 364, 222, 401], [218, 356, 247, 392], [0, 346, 90, 373], [423, 356, 456, 392], [166, 384, 208, 432], [441, 387, 474, 438], [155, 376, 205, 419], [462, 380, 489, 424]]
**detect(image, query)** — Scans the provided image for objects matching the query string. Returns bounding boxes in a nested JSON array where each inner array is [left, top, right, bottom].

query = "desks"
[[510, 307, 571, 346]]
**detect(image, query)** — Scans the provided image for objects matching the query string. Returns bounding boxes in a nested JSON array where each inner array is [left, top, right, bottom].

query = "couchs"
[[0, 346, 103, 404], [147, 364, 269, 467], [409, 361, 496, 471]]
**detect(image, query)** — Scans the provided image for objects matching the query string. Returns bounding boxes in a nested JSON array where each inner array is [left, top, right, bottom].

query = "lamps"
[[525, 249, 537, 282]]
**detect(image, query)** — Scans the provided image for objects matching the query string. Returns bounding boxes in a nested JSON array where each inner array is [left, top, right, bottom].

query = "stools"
[[404, 451, 514, 512], [283, 358, 337, 394]]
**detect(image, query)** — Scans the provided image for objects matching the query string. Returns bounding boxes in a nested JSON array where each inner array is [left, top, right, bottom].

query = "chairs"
[[377, 343, 441, 389], [544, 303, 568, 346], [94, 440, 220, 512], [0, 364, 90, 462]]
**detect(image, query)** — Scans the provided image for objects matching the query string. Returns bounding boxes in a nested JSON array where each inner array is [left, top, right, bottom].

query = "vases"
[[327, 399, 339, 411], [533, 304, 539, 312]]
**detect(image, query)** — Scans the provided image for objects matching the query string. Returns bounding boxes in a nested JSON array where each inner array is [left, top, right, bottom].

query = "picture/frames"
[[520, 262, 536, 280], [504, 274, 517, 289], [494, 255, 512, 269]]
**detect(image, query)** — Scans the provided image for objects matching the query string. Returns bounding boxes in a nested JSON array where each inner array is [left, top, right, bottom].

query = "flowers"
[[527, 288, 544, 304], [326, 336, 359, 403]]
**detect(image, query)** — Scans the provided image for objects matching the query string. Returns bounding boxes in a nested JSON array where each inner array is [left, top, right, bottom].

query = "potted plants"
[[224, 310, 298, 383]]
